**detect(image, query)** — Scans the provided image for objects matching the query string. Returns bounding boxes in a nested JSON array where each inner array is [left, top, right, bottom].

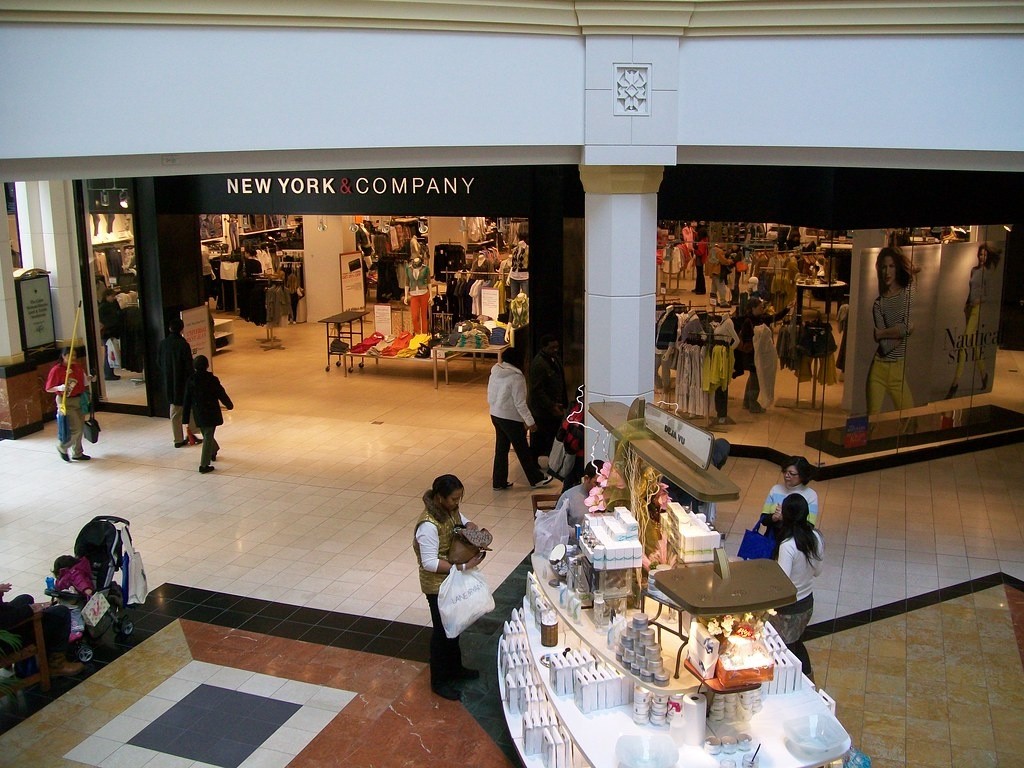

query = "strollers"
[[43, 514, 135, 663]]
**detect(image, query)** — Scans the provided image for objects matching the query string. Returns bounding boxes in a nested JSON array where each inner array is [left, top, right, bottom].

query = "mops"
[[56, 300, 81, 444]]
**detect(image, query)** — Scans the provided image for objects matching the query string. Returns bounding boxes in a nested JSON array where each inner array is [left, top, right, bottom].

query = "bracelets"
[[462, 564, 466, 571]]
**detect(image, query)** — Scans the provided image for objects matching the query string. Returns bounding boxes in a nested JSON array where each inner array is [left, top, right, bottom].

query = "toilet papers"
[[683, 692, 708, 745]]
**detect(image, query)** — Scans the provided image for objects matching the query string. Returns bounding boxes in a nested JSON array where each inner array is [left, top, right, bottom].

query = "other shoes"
[[199, 465, 214, 473], [105, 375, 121, 381], [720, 305, 732, 308], [174, 439, 187, 448], [692, 289, 706, 295], [729, 300, 739, 305], [57, 448, 69, 461], [211, 453, 216, 462], [72, 454, 91, 460], [187, 435, 202, 443], [945, 383, 958, 398], [981, 373, 988, 390]]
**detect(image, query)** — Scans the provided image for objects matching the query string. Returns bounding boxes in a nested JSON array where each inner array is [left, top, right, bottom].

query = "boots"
[[48, 654, 84, 677]]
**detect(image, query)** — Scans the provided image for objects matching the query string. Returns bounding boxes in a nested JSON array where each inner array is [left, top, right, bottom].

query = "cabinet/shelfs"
[[495, 398, 873, 768], [91, 212, 941, 410]]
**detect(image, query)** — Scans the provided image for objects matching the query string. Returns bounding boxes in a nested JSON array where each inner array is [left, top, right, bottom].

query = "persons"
[[747, 277, 762, 305], [156, 318, 203, 448], [234, 247, 262, 320], [127, 254, 136, 276], [201, 244, 216, 305], [413, 474, 486, 700], [487, 334, 568, 491], [739, 297, 796, 413], [0, 555, 95, 687], [691, 228, 743, 308], [182, 355, 234, 474], [654, 305, 740, 424], [45, 346, 96, 461], [941, 244, 1002, 400], [356, 223, 375, 269], [403, 235, 434, 334], [554, 459, 605, 546], [866, 247, 921, 415], [281, 255, 299, 325], [504, 293, 529, 348], [802, 240, 822, 252], [760, 455, 825, 685], [472, 240, 529, 321], [98, 289, 122, 380]]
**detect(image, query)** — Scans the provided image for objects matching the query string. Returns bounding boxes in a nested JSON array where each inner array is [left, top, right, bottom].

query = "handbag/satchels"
[[736, 515, 775, 560], [120, 527, 148, 607], [84, 417, 101, 444], [448, 528, 493, 563], [81, 592, 111, 626], [438, 565, 496, 637], [105, 337, 121, 368], [238, 260, 247, 280]]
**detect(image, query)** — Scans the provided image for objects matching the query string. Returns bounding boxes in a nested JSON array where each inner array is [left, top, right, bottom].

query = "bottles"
[[669, 712, 684, 746], [577, 566, 591, 594], [46, 577, 55, 590], [543, 566, 547, 578], [594, 591, 605, 633]]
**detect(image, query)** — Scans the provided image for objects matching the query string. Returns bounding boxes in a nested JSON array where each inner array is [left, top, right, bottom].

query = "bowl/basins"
[[615, 733, 679, 768], [742, 754, 760, 768], [784, 714, 848, 752], [720, 758, 736, 768]]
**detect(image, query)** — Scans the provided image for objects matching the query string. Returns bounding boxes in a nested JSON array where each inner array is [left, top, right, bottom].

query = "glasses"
[[782, 471, 799, 476]]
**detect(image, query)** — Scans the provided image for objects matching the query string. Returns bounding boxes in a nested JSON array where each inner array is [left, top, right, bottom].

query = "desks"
[[432, 342, 511, 388], [342, 349, 477, 385], [317, 311, 370, 374]]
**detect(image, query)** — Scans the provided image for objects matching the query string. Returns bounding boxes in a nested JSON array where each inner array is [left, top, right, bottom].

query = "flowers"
[[584, 462, 672, 566], [698, 608, 778, 655]]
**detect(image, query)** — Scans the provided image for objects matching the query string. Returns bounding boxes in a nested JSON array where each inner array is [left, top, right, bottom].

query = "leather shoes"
[[432, 684, 462, 700], [453, 668, 479, 680]]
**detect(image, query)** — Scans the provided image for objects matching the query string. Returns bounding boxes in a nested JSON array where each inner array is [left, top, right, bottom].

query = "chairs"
[[0, 612, 51, 698], [532, 494, 561, 520]]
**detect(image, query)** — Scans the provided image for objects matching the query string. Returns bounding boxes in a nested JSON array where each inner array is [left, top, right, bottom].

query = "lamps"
[[101, 190, 110, 207], [119, 192, 128, 209], [348, 216, 359, 232], [378, 216, 390, 233], [418, 216, 428, 234], [317, 215, 327, 232]]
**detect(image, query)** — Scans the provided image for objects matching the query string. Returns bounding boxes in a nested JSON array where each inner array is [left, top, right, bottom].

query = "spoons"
[[748, 744, 762, 767]]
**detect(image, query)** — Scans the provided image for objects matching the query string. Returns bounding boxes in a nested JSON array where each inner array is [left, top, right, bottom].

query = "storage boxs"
[[658, 502, 721, 562], [578, 506, 643, 571]]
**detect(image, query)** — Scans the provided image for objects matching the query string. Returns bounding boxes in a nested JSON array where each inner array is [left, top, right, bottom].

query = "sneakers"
[[531, 475, 553, 488], [492, 482, 514, 490]]
